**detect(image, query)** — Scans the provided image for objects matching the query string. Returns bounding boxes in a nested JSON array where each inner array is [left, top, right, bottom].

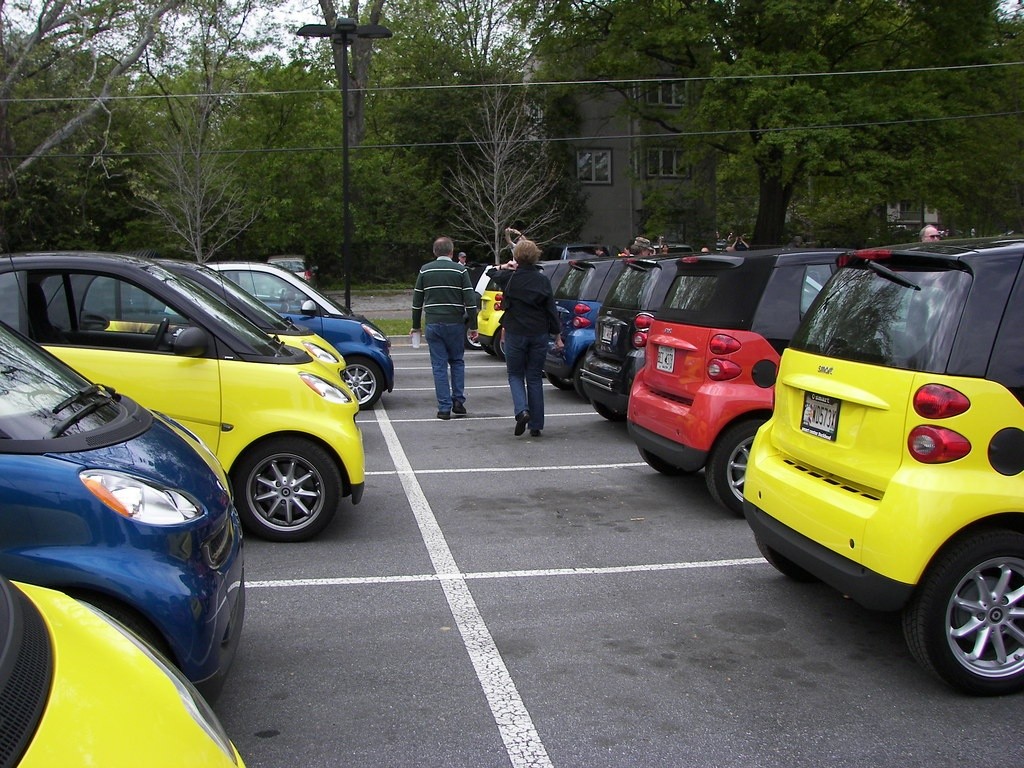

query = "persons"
[[614, 236, 656, 309], [701, 247, 709, 254], [409, 237, 479, 419], [593, 245, 611, 257], [484, 239, 565, 436], [661, 243, 671, 254], [457, 251, 469, 268], [919, 224, 943, 243], [724, 231, 750, 252]]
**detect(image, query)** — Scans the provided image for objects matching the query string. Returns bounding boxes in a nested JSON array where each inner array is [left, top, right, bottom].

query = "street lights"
[[294, 18, 393, 312]]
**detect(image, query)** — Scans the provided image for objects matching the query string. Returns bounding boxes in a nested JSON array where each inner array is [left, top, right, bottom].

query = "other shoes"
[[515, 409, 529, 436], [530, 429, 541, 437], [452, 399, 466, 414], [437, 410, 451, 420]]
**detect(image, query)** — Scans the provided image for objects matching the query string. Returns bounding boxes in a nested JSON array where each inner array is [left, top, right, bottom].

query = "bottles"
[[412, 332, 420, 348]]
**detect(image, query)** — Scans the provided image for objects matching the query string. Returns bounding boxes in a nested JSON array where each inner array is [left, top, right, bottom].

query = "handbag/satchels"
[[500, 272, 515, 310]]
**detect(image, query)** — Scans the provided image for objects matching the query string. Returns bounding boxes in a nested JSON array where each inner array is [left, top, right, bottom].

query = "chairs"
[[27, 282, 69, 343]]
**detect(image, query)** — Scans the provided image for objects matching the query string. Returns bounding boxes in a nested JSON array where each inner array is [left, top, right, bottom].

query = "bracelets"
[[499, 264, 502, 270]]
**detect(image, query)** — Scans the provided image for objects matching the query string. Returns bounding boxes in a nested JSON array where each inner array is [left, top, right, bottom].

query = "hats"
[[459, 252, 466, 258]]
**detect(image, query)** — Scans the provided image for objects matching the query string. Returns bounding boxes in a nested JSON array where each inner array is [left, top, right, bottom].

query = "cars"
[[1, 320, 246, 688], [152, 255, 393, 410], [1, 571, 250, 766], [0, 250, 365, 542], [741, 235, 1024, 697], [466, 242, 712, 420], [625, 249, 855, 515]]
